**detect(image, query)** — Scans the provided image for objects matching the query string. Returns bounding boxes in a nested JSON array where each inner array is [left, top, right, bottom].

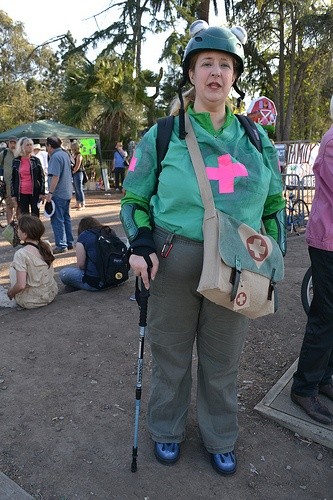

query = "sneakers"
[[154, 442, 180, 465], [213, 451, 237, 476]]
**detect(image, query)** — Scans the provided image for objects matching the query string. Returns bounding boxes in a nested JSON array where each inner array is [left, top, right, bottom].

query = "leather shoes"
[[319, 381, 333, 401], [291, 392, 333, 424]]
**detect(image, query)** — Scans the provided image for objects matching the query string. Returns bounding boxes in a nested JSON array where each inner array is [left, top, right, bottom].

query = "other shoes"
[[71, 206, 85, 211], [65, 284, 78, 292], [67, 246, 73, 250], [51, 246, 65, 255]]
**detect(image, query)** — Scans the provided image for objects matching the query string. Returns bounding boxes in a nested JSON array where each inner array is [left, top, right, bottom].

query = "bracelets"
[[49, 191, 53, 194]]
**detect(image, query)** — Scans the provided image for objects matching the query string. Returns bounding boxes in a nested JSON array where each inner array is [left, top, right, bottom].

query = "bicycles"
[[300, 265, 314, 318], [281, 192, 310, 236], [0, 179, 15, 228]]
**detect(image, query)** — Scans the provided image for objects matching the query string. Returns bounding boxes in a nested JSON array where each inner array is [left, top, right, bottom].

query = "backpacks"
[[82, 225, 130, 290]]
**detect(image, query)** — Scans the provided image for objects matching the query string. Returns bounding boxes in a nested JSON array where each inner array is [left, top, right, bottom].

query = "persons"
[[59, 217, 126, 292], [70, 141, 85, 211], [0, 136, 49, 225], [6, 214, 59, 310], [88, 166, 97, 182], [112, 142, 128, 191], [120, 19, 288, 477], [44, 135, 74, 253], [290, 95, 333, 424]]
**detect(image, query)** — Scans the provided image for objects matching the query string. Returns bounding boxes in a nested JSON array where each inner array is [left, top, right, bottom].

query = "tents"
[[0, 118, 100, 183]]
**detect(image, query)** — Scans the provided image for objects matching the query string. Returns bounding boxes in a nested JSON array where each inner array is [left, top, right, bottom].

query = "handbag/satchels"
[[124, 160, 130, 166], [2, 208, 20, 247], [196, 207, 285, 318], [83, 166, 89, 184], [0, 181, 6, 197]]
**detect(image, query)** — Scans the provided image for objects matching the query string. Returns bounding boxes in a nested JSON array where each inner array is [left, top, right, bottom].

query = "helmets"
[[182, 20, 248, 73]]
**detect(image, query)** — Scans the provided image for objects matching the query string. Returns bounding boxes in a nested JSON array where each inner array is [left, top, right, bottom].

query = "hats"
[[45, 200, 55, 218], [34, 144, 45, 151]]
